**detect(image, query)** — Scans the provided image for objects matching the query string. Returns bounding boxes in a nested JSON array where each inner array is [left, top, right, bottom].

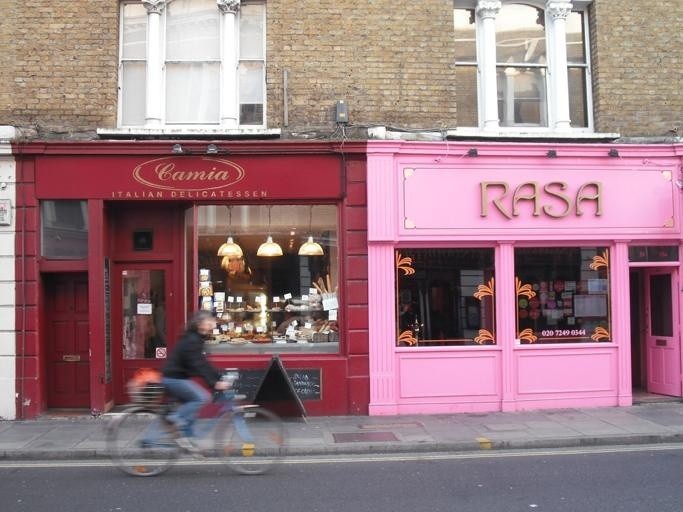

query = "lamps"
[[206, 144, 218, 155], [256, 205, 284, 257], [217, 206, 244, 256], [298, 205, 324, 256], [171, 143, 184, 154]]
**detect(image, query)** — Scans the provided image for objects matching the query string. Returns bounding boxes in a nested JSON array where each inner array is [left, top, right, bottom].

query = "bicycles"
[[105, 376, 284, 478]]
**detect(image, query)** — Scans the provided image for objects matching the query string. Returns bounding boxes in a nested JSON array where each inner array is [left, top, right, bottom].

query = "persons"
[[161, 314, 232, 454]]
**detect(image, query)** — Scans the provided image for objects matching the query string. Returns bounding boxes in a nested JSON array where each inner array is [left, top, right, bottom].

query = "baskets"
[[307, 333, 338, 343], [127, 381, 166, 408]]
